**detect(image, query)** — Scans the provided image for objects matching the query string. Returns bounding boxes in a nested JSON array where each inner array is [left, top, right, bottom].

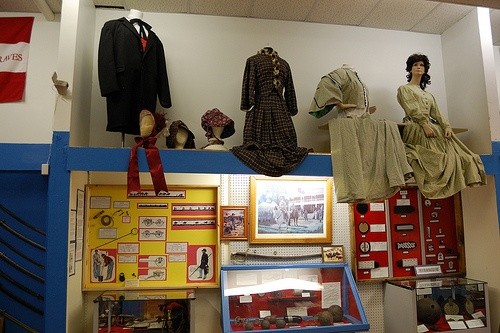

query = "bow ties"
[[130, 18, 152, 38]]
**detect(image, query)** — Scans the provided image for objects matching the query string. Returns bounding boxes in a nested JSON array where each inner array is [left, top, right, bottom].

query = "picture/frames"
[[250, 176, 333, 244], [219, 206, 248, 241], [321, 245, 345, 263]]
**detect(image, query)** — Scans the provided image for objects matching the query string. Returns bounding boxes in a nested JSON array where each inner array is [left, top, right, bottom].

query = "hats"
[[140, 110, 166, 136], [201, 108, 235, 139], [166, 120, 195, 149]]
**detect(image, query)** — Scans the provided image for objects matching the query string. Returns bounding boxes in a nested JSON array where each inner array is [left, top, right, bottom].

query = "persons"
[[304, 210, 309, 221], [135, 109, 167, 147], [166, 120, 196, 149], [308, 64, 413, 204], [231, 47, 313, 177], [201, 108, 236, 150], [397, 54, 488, 199], [98, 8, 172, 135], [293, 209, 298, 226]]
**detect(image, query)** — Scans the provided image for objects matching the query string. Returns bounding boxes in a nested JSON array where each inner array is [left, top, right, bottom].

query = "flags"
[[0, 16, 35, 103]]
